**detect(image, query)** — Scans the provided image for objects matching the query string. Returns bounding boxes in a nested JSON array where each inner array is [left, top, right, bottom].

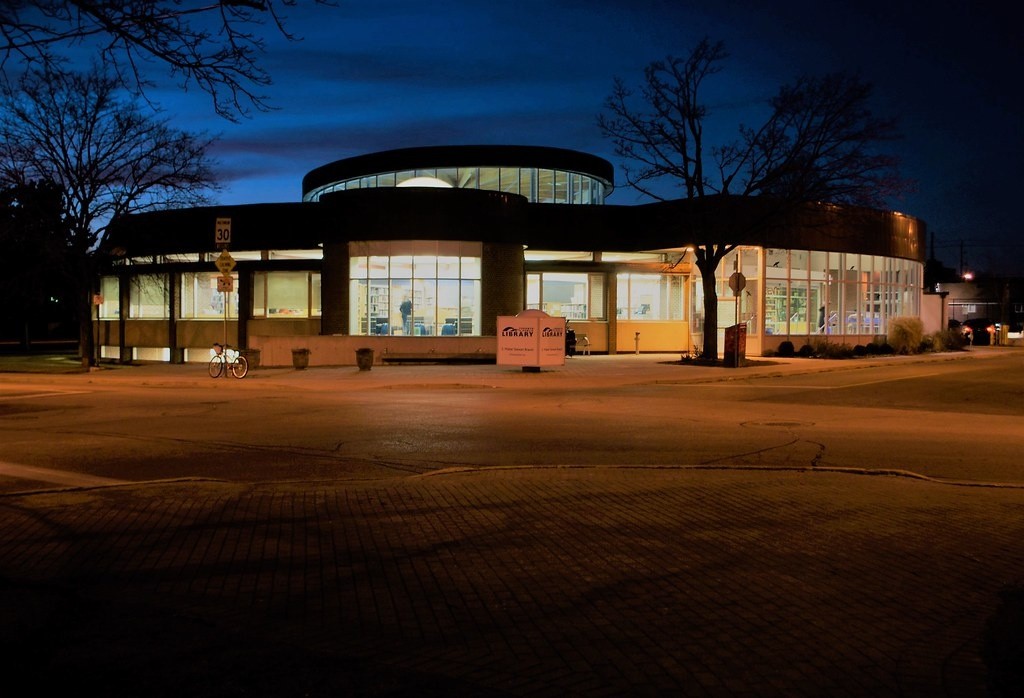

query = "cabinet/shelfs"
[[358, 284, 425, 335], [765, 288, 817, 333]]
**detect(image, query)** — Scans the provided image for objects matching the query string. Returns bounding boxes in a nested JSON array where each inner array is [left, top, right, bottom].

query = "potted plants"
[[355, 347, 374, 372], [239, 348, 261, 368], [290, 348, 312, 370]]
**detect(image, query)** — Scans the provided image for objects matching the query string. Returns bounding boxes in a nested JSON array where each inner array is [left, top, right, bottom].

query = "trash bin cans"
[[724, 322, 747, 367]]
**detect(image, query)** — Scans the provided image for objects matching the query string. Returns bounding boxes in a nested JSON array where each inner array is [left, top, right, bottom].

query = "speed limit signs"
[[216, 218, 231, 244]]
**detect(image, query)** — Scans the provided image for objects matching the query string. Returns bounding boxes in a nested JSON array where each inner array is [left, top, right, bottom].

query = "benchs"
[[574, 333, 591, 356]]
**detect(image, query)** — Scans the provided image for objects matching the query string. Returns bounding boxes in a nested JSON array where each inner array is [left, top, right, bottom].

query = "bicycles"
[[208, 343, 249, 379]]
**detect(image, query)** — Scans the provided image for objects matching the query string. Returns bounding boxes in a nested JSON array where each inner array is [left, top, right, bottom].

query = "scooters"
[[566, 320, 577, 356]]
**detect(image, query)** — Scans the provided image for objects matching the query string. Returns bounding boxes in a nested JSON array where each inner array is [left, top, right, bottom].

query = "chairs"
[[830, 312, 881, 334]]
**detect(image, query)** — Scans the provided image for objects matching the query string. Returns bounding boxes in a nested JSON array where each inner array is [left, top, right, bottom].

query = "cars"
[[966, 318, 1001, 345]]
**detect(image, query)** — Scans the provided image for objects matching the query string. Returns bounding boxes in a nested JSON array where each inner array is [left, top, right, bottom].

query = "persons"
[[819, 300, 830, 331], [400, 296, 411, 331]]
